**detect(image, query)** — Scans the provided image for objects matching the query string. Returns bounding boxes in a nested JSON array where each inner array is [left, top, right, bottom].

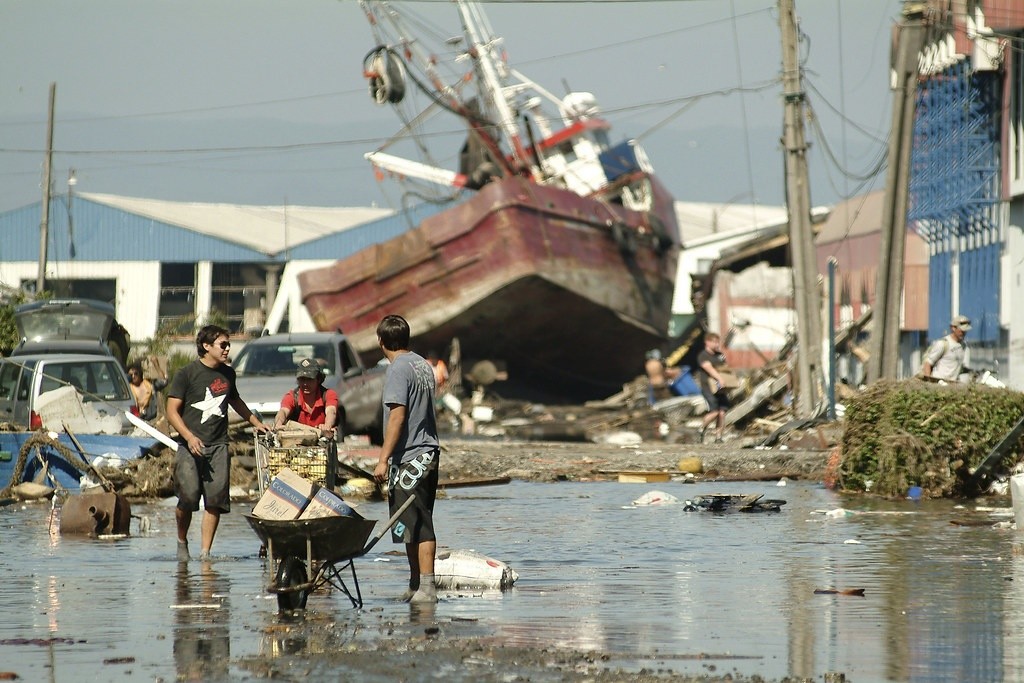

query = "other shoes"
[[694, 429, 704, 444], [715, 439, 723, 443], [176, 538, 190, 565]]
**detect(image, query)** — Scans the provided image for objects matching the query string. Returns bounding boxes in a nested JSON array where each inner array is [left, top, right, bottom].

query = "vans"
[[1, 352, 137, 437]]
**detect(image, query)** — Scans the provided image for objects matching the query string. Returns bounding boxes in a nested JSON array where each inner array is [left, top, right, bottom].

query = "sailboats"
[[296, 0, 681, 407]]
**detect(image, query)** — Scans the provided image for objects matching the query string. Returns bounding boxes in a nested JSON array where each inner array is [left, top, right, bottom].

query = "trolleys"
[[241, 494, 417, 611], [252, 429, 338, 497]]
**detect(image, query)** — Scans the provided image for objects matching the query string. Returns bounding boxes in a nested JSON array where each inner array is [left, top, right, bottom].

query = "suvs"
[[222, 327, 365, 425]]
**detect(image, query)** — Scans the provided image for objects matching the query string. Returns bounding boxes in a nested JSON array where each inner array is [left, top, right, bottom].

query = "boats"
[[0, 429, 158, 503]]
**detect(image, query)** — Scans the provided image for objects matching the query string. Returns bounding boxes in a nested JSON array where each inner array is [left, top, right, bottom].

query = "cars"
[[10, 295, 130, 369]]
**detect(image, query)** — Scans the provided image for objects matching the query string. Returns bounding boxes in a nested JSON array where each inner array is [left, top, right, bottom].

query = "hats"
[[952, 315, 972, 331], [296, 359, 321, 379]]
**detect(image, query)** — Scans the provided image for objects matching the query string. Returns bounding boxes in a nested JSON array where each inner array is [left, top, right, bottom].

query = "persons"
[[274, 358, 339, 431], [425, 349, 449, 405], [166, 325, 272, 558], [374, 314, 441, 603], [126, 362, 156, 413], [644, 348, 672, 404], [924, 315, 973, 386], [696, 332, 730, 435]]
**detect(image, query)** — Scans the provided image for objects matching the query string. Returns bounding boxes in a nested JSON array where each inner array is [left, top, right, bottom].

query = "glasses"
[[210, 341, 230, 350], [960, 320, 971, 325]]
